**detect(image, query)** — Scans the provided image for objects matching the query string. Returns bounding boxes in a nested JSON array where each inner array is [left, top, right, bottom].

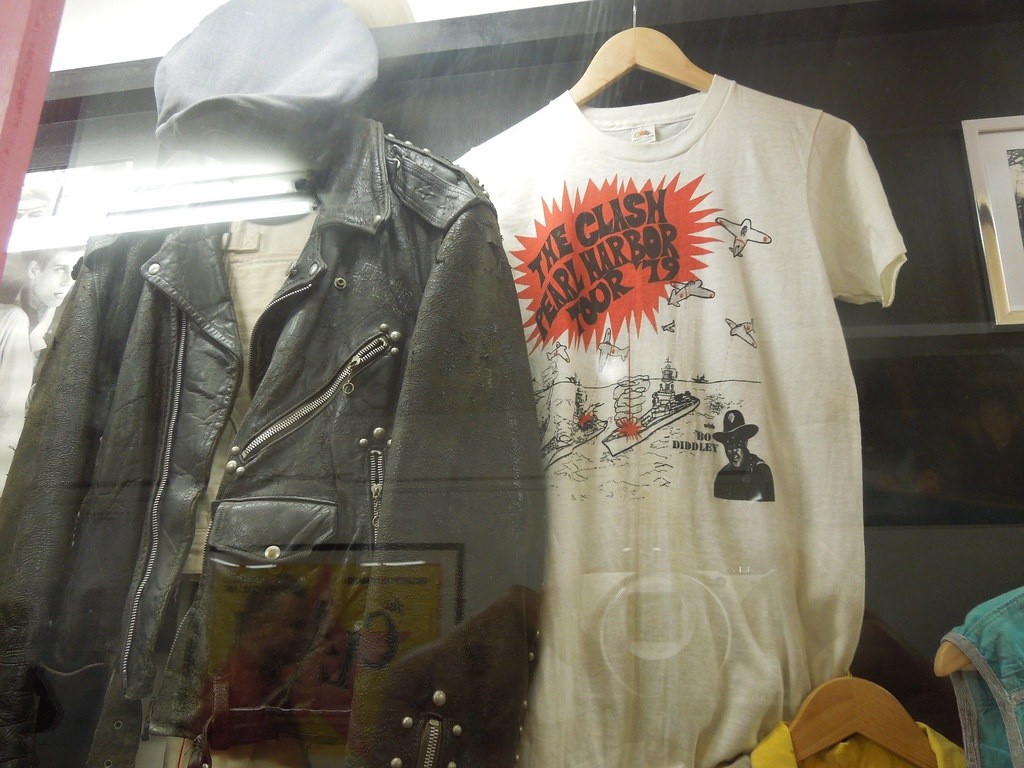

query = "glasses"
[[725, 441, 746, 450]]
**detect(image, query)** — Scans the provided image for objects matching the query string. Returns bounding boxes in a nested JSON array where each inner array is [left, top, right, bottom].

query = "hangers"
[[787, 677, 938, 768], [569, 1, 714, 105]]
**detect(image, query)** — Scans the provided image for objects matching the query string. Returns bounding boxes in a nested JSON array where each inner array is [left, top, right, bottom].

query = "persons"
[[712, 410, 775, 502]]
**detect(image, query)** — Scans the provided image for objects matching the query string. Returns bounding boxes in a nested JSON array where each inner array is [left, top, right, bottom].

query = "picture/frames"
[[960, 114, 1024, 326], [205, 542, 466, 745]]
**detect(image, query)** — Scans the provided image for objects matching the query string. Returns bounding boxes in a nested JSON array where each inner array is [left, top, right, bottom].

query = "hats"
[[154, 0, 379, 146], [713, 410, 759, 442]]
